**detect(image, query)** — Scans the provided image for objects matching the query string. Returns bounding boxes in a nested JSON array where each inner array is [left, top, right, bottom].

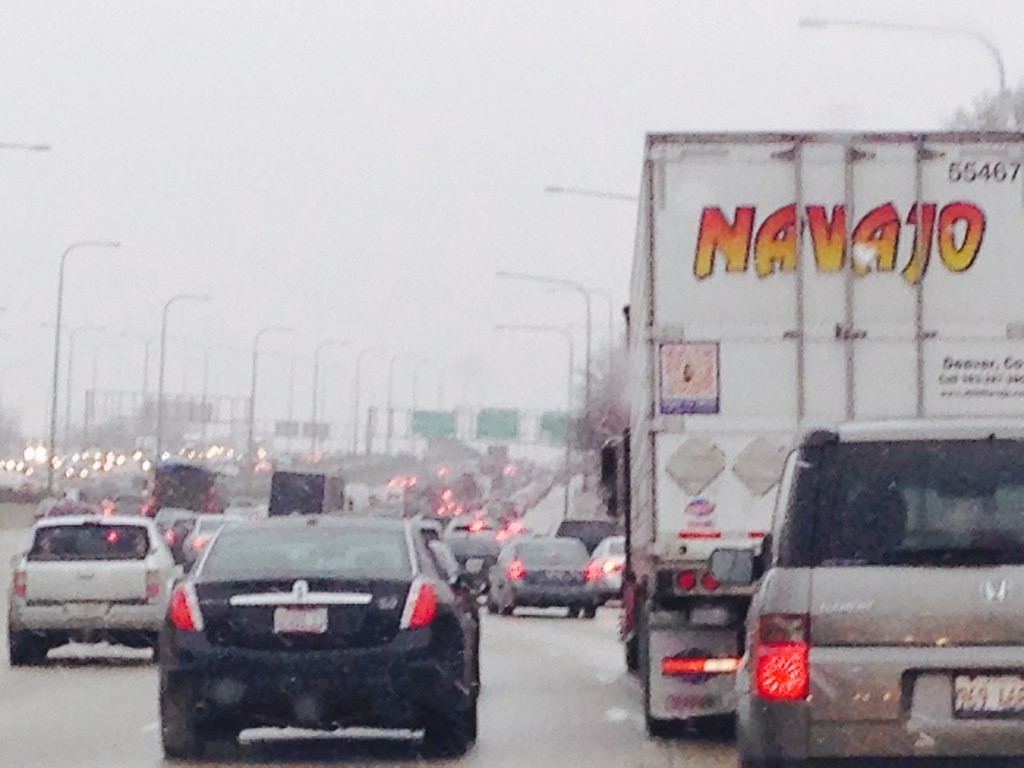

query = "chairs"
[[857, 489, 908, 545], [50, 535, 137, 552]]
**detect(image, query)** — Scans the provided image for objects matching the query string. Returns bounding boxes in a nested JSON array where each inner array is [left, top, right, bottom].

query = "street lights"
[[546, 186, 638, 200], [47, 239, 121, 488], [797, 15, 1006, 124], [494, 322, 574, 515], [155, 292, 210, 462], [496, 271, 592, 491], [0, 142, 51, 151], [246, 324, 298, 454]]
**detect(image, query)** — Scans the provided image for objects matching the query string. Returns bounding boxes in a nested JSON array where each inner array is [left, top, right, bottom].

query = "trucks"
[[599, 129, 1024, 728], [145, 464, 230, 517], [268, 472, 354, 517]]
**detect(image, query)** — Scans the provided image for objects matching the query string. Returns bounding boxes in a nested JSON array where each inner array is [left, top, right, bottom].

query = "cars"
[[487, 534, 595, 616], [735, 422, 1024, 768], [11, 515, 184, 664], [158, 515, 481, 754], [590, 536, 626, 605]]
